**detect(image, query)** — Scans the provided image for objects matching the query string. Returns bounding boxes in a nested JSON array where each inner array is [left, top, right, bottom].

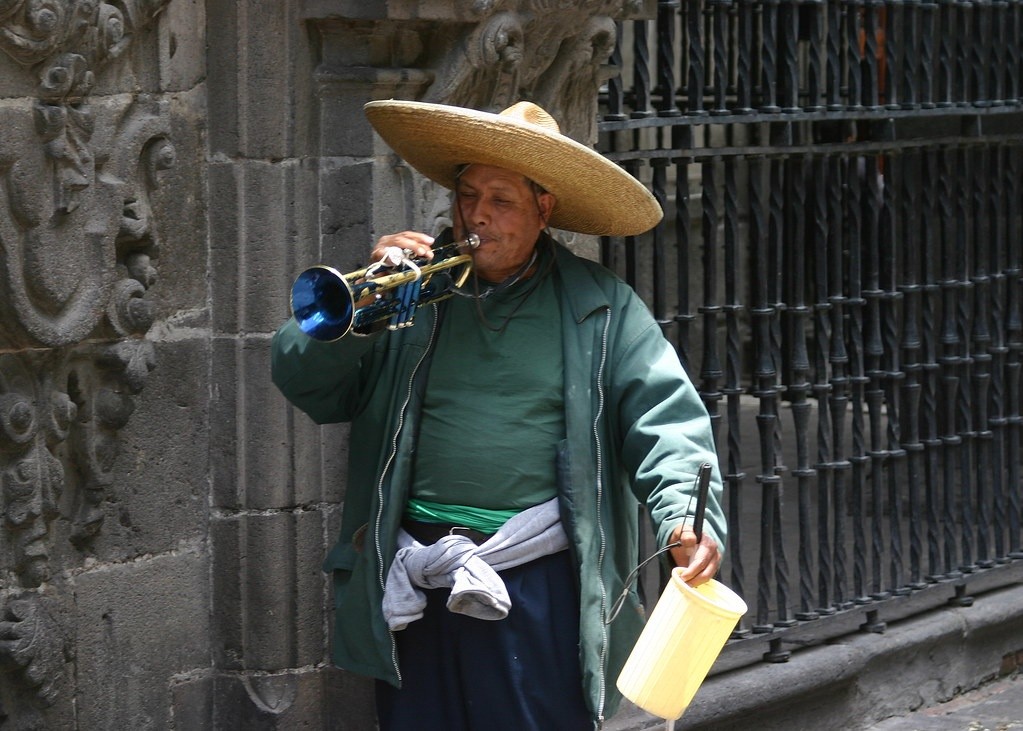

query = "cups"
[[615, 567, 748, 720]]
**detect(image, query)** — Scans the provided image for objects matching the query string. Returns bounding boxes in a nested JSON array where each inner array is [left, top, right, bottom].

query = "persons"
[[271, 100, 728, 731]]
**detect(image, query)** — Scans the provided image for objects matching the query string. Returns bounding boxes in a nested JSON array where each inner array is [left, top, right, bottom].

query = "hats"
[[364, 100, 664, 237]]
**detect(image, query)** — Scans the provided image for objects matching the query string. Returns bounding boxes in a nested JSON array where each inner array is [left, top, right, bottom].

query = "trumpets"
[[290, 234, 480, 344]]
